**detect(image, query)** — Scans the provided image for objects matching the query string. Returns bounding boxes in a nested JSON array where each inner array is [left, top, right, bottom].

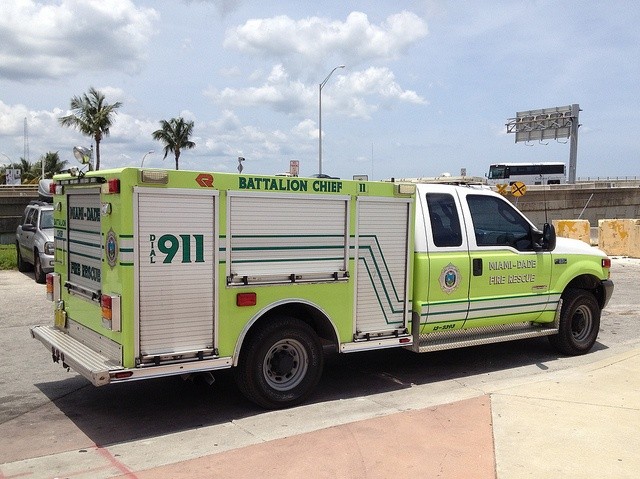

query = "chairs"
[[448, 218, 463, 242], [426, 212, 445, 247]]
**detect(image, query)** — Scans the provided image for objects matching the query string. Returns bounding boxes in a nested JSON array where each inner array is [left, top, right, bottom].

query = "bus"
[[485, 161, 567, 187]]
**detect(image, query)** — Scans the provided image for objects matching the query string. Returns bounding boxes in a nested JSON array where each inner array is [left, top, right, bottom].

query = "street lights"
[[141, 151, 154, 167], [317, 64, 348, 175]]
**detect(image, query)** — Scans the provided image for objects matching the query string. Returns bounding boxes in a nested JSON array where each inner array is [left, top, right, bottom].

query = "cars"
[[273, 172, 299, 177], [309, 173, 340, 180]]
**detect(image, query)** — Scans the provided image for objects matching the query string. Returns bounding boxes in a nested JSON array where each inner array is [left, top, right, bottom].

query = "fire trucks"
[[29, 145, 614, 410]]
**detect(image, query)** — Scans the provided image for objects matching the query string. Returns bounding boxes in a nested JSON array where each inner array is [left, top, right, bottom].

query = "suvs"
[[15, 200, 54, 286]]
